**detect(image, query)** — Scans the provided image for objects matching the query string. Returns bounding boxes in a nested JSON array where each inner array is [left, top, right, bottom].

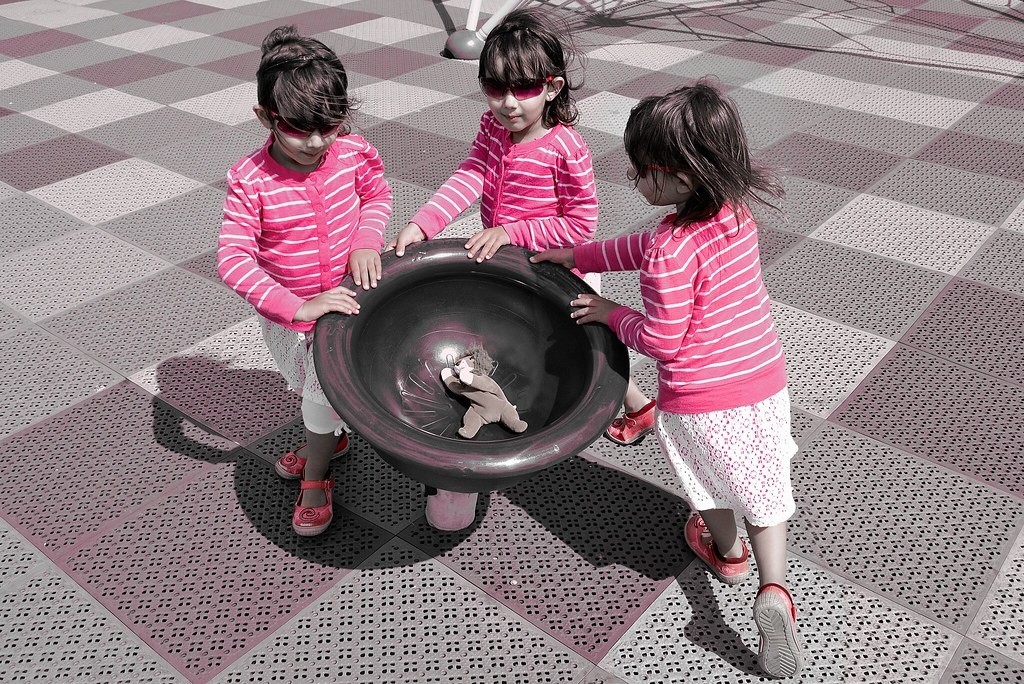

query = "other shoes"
[[292, 467, 335, 536], [605, 399, 657, 445], [684, 515, 749, 584], [274, 435, 350, 479], [753, 583, 804, 678]]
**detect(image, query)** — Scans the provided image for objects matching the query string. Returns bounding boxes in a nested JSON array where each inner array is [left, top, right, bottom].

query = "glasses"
[[481, 74, 556, 100], [627, 164, 695, 181], [264, 107, 346, 138]]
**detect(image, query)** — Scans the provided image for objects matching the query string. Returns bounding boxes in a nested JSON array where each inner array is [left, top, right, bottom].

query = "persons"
[[385, 7, 656, 446], [529, 74, 804, 677], [217, 24, 395, 536]]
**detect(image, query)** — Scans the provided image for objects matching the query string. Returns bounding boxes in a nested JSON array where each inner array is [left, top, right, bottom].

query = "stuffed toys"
[[442, 347, 528, 439]]
